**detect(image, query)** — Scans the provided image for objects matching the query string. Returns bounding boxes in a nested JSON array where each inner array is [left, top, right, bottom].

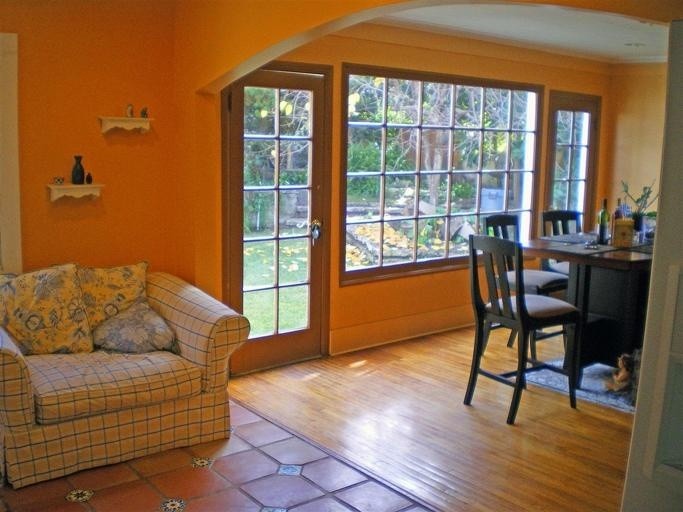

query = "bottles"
[[597, 197, 609, 244], [612, 198, 623, 240]]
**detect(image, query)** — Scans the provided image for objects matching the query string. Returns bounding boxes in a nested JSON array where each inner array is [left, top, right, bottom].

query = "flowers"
[[619, 177, 661, 212]]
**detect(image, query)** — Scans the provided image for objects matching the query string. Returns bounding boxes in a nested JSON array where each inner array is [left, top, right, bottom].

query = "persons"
[[604, 352, 634, 394]]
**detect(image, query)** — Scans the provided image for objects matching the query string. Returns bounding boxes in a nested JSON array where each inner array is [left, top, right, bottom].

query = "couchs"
[[0, 270, 251, 488]]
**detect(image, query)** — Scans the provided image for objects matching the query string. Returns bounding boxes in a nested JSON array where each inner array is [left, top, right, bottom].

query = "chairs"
[[482, 215, 572, 347], [462, 235, 582, 425], [539, 210, 585, 277]]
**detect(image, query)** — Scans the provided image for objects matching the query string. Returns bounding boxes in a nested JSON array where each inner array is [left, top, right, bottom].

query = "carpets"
[[519, 355, 638, 415]]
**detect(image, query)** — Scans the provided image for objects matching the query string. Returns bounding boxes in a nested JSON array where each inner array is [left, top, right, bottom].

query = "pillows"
[[0, 262, 95, 352], [94, 295, 178, 354], [77, 259, 151, 330]]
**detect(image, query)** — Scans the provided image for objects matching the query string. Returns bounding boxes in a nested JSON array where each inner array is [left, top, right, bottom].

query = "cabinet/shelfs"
[[97, 116, 153, 134], [47, 183, 104, 203]]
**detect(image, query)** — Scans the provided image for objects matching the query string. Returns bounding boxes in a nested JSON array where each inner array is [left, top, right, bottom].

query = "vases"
[[72, 155, 84, 184], [630, 217, 643, 243]]
